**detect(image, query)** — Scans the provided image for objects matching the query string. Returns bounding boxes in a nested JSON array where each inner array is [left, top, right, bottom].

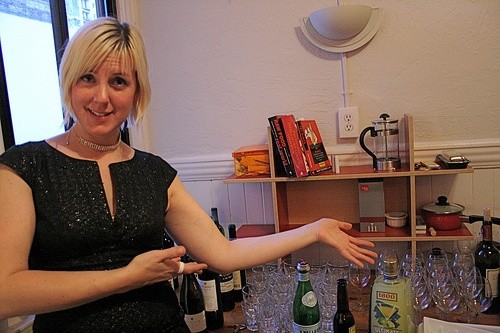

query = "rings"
[[178, 262, 185, 274]]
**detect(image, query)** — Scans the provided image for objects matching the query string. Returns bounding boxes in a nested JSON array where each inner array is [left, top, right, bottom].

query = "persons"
[[0, 18, 378, 333]]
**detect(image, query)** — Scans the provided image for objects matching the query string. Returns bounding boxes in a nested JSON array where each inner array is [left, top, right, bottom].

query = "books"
[[269, 113, 332, 180]]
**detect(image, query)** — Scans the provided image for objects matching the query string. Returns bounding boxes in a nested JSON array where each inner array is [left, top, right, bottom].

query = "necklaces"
[[66, 125, 122, 151]]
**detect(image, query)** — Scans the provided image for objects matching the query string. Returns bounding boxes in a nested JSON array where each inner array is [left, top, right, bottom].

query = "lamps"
[[299, 4, 382, 53]]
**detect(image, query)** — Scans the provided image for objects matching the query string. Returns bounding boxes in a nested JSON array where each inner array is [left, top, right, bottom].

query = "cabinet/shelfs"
[[224, 113, 474, 275], [358, 177, 385, 233]]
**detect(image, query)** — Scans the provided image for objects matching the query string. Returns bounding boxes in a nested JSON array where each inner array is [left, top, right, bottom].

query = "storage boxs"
[[416, 214, 427, 235], [232, 145, 270, 177]]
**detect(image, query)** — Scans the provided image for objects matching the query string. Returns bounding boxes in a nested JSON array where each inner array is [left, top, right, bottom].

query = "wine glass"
[[347, 241, 493, 324], [240, 258, 372, 333]]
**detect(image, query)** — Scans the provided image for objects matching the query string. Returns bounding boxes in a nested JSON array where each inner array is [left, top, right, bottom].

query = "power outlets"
[[338, 106, 359, 139]]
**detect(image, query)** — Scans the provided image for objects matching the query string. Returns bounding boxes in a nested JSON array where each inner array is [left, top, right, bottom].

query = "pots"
[[420, 196, 500, 231]]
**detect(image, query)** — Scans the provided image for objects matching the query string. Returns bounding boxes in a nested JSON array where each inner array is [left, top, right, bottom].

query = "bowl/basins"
[[385, 212, 408, 228], [437, 150, 472, 169]]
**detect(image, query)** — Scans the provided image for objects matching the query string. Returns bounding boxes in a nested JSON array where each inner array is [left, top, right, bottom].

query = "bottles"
[[368, 258, 416, 333], [332, 278, 356, 333], [474, 208, 500, 316], [162, 208, 249, 333], [292, 260, 323, 333]]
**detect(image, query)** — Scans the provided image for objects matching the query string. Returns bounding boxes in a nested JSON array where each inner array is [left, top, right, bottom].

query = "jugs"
[[359, 113, 402, 172]]
